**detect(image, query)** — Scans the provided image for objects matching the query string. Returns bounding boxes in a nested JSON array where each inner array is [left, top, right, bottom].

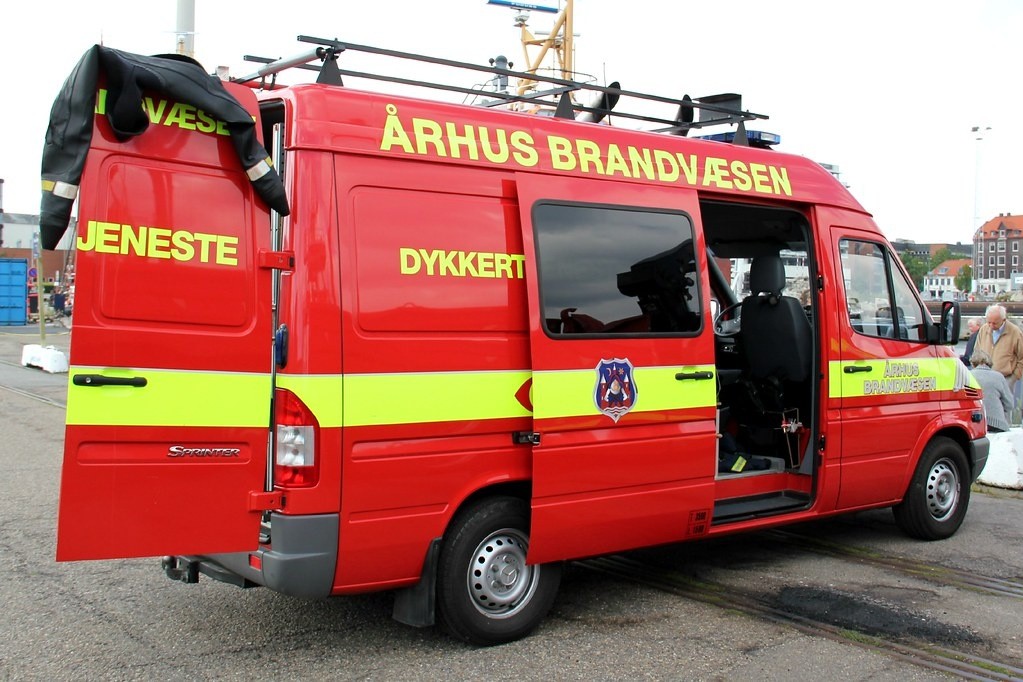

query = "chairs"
[[740, 249, 812, 384]]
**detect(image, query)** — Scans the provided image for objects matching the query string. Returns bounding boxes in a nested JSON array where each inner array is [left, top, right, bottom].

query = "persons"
[[875, 300, 907, 338], [964, 319, 981, 359], [848, 299, 863, 332], [800, 291, 811, 306], [972, 304, 1023, 408], [969, 350, 1015, 434]]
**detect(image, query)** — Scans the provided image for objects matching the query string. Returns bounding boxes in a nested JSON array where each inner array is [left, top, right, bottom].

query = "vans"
[[55, 34, 995, 650]]
